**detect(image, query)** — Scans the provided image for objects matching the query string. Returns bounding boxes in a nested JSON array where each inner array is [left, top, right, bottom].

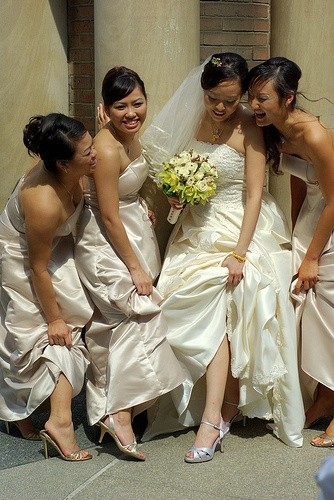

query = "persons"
[[0, 52, 334, 463]]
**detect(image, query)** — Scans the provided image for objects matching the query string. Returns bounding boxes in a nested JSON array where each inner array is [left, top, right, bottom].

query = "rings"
[[238, 280, 241, 281]]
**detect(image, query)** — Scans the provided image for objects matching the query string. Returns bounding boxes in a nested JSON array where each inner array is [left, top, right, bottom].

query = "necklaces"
[[58, 182, 77, 202], [210, 117, 232, 142], [280, 109, 301, 143], [109, 124, 130, 155]]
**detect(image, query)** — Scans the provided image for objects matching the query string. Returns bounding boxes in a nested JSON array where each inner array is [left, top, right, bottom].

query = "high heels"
[[223, 401, 246, 427], [304, 406, 334, 429], [97, 415, 146, 461], [3, 420, 43, 441], [184, 417, 230, 463], [310, 417, 334, 447], [39, 429, 93, 461]]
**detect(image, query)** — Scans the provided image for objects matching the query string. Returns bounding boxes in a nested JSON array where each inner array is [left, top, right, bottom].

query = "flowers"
[[152, 149, 220, 224], [211, 57, 222, 67]]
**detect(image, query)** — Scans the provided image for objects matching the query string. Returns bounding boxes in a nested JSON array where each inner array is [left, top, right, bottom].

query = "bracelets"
[[231, 252, 246, 263]]
